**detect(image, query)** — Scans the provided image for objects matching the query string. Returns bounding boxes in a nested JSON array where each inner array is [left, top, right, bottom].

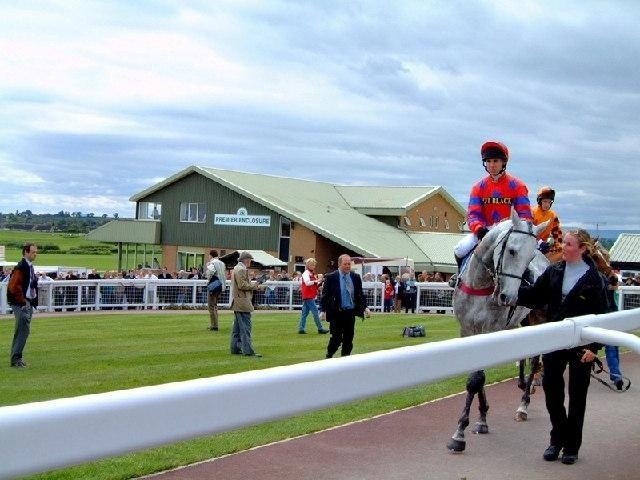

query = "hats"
[[239, 251, 254, 260]]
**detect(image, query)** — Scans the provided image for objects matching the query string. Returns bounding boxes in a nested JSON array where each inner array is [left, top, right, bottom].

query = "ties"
[[343, 273, 353, 304]]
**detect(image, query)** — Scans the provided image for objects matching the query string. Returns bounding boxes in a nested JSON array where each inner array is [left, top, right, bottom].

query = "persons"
[[36, 256, 206, 312], [252, 257, 330, 334], [530, 186, 564, 253], [6, 243, 38, 366], [229, 251, 263, 357], [206, 250, 234, 331], [449, 141, 531, 290], [318, 253, 372, 359], [362, 266, 447, 314], [1, 269, 14, 314], [151, 207, 158, 219], [519, 228, 639, 465]]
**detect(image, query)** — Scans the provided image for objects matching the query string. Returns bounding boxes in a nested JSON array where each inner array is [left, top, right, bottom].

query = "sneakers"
[[299, 331, 308, 334], [559, 450, 578, 465], [543, 443, 562, 460], [318, 329, 329, 334], [245, 351, 263, 357], [207, 326, 219, 332]]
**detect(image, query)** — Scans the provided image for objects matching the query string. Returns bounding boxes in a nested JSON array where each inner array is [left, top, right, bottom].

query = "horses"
[[448, 204, 552, 451], [521, 234, 619, 386]]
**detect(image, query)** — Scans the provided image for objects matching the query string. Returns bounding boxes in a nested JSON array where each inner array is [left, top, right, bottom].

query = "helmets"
[[536, 187, 555, 206], [481, 140, 509, 164]]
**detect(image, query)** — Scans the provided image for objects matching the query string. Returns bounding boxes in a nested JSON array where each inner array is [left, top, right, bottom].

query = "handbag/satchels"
[[402, 325, 426, 337]]
